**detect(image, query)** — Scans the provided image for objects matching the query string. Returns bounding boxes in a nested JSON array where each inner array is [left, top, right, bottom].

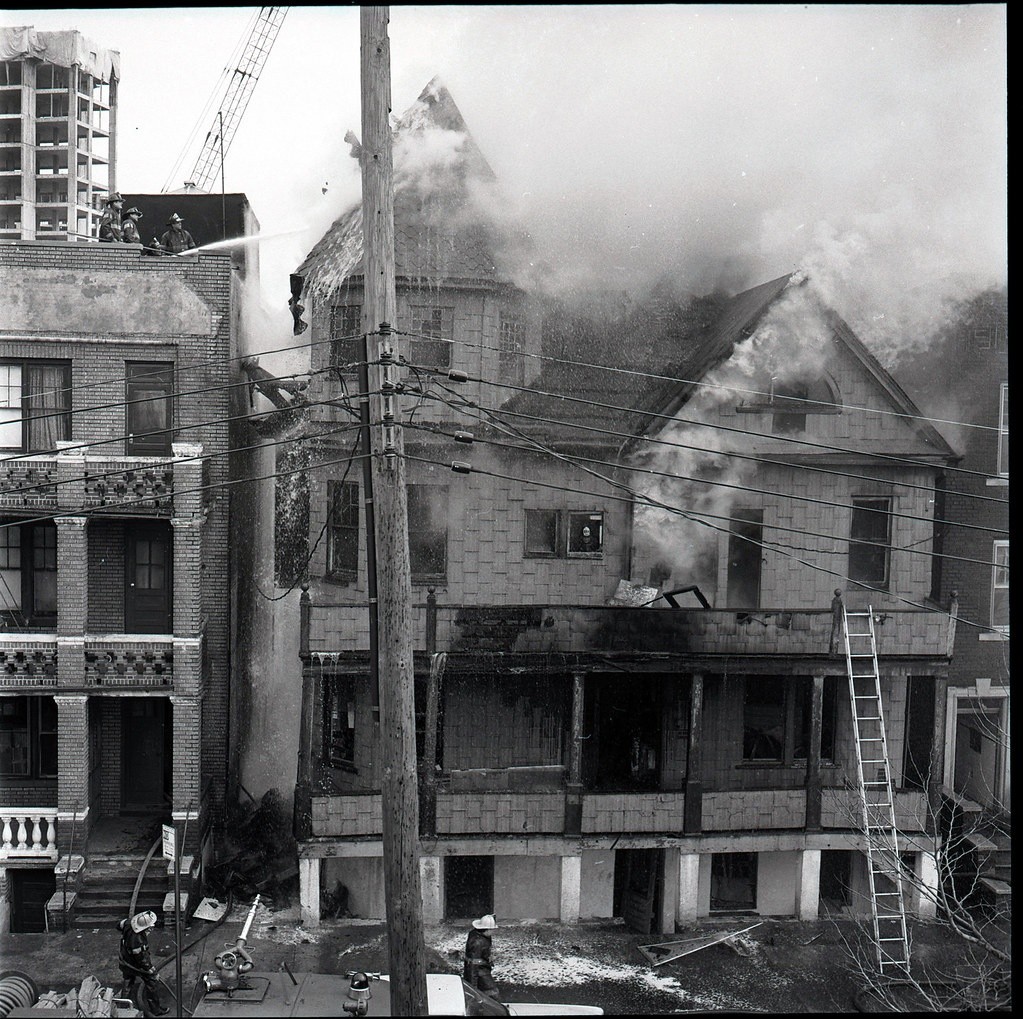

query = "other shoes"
[[152, 1007, 170, 1016]]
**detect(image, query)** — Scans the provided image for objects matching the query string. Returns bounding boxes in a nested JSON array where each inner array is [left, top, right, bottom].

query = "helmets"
[[131, 910, 157, 933], [472, 913, 498, 929]]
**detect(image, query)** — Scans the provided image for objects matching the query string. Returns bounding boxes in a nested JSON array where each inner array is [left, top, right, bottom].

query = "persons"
[[115, 911, 170, 1016], [145, 213, 196, 256], [464, 915, 499, 1001], [99, 193, 141, 243]]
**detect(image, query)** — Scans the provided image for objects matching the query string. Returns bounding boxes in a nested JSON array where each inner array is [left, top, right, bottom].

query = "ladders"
[[837, 603, 916, 978]]
